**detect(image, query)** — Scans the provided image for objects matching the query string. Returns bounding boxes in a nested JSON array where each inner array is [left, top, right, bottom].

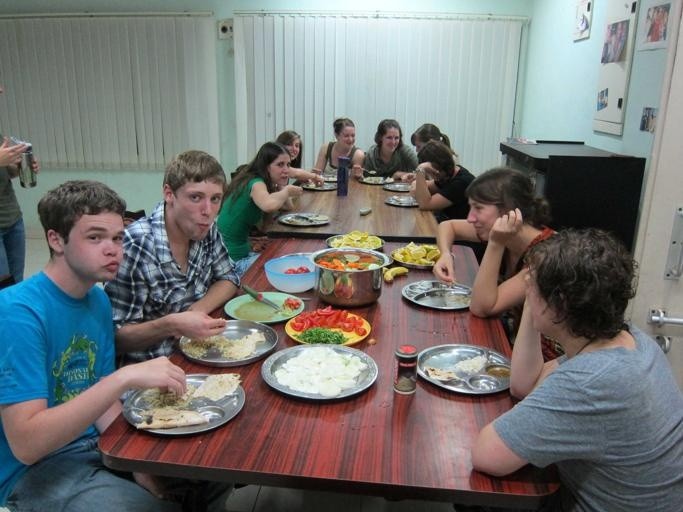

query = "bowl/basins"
[[264, 254, 316, 295]]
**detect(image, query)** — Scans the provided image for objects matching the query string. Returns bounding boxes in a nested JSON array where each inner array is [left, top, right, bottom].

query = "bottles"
[[15, 144, 36, 188], [337, 157, 348, 195]]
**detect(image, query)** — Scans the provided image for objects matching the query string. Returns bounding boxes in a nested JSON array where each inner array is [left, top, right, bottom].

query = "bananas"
[[384, 266, 409, 283]]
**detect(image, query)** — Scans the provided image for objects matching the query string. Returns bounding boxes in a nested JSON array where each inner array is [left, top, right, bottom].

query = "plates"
[[388, 247, 456, 271], [177, 318, 279, 367], [223, 290, 304, 324], [284, 311, 370, 345], [301, 172, 417, 208], [260, 345, 379, 401], [324, 234, 386, 252]]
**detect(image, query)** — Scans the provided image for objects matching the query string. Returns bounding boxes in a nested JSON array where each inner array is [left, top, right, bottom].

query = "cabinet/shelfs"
[[496, 140, 646, 256]]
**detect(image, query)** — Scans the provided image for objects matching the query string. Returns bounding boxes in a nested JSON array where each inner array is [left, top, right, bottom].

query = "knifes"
[[242, 284, 279, 308]]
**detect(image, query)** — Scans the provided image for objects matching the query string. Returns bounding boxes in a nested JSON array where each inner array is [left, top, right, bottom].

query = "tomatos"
[[285, 298, 368, 337], [285, 268, 297, 274], [297, 266, 309, 273]]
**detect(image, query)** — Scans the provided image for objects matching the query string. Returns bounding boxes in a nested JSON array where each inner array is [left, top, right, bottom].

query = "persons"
[[602, 22, 628, 61], [643, 6, 670, 41]]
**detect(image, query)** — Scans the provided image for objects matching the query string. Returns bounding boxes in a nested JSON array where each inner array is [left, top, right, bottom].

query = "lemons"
[[392, 241, 440, 265], [330, 230, 382, 249]]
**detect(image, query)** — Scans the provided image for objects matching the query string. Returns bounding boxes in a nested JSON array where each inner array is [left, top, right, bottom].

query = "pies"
[[137, 407, 209, 430]]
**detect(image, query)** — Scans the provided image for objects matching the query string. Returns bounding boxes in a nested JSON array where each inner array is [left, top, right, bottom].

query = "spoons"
[[360, 168, 375, 174]]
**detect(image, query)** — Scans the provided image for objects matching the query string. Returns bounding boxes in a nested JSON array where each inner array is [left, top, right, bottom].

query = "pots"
[[312, 248, 393, 307]]
